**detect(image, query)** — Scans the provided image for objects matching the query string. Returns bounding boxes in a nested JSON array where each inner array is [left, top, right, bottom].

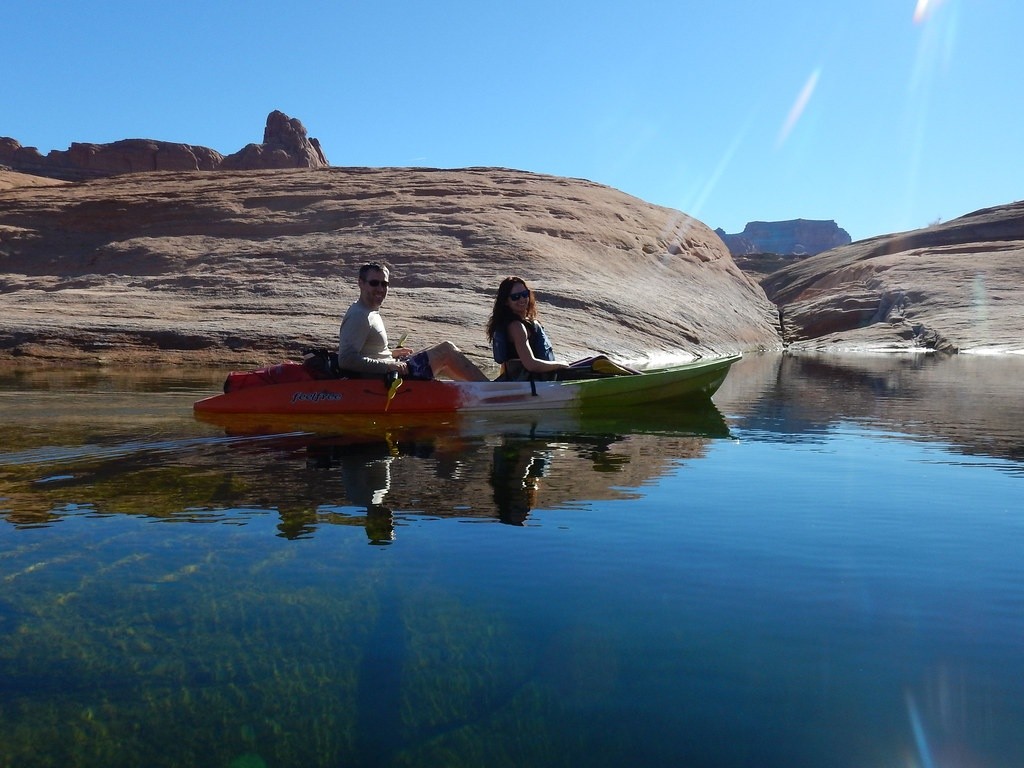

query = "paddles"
[[387, 332, 409, 401]]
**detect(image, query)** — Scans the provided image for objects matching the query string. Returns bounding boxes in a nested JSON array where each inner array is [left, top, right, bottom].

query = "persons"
[[487, 276, 646, 375], [338, 262, 490, 382], [492, 443, 560, 527], [341, 439, 436, 540]]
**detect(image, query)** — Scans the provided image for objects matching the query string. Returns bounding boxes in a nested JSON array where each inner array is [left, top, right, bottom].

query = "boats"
[[199, 352, 745, 415]]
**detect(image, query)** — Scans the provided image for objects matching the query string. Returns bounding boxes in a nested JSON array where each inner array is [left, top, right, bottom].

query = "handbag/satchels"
[[306, 350, 340, 381]]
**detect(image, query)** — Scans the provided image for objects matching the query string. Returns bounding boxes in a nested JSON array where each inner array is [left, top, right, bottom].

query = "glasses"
[[507, 290, 529, 303], [368, 279, 390, 289]]
